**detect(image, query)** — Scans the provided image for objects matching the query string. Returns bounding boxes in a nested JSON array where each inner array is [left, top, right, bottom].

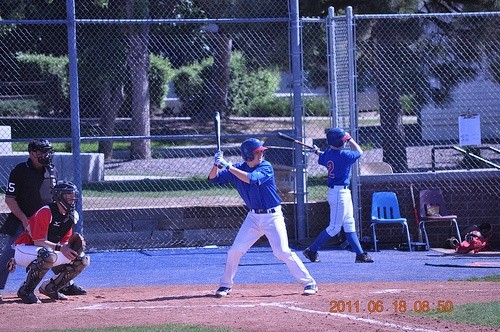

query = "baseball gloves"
[[67, 232, 86, 262]]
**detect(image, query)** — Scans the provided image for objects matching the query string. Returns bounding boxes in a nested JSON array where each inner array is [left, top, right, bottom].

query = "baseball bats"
[[213, 111, 224, 169], [276, 130, 316, 150]]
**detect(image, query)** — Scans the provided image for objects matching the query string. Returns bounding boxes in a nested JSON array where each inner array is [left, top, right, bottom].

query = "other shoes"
[[354, 251, 374, 263], [39, 279, 69, 301], [216, 286, 232, 298], [303, 284, 318, 294], [17, 285, 43, 305], [59, 283, 85, 295], [303, 248, 320, 263]]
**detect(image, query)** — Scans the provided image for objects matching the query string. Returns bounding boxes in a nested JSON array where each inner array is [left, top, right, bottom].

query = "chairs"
[[370, 191, 412, 252], [418, 190, 463, 251]]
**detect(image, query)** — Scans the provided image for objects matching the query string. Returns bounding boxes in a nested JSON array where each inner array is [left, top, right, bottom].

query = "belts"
[[328, 185, 348, 189], [248, 208, 275, 214]]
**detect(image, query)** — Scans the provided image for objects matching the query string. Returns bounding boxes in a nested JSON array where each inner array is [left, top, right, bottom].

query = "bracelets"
[[55, 244, 58, 251], [58, 245, 61, 251], [225, 161, 233, 171]]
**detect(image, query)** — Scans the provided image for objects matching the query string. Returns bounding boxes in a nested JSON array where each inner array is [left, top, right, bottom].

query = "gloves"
[[343, 132, 351, 143], [214, 150, 232, 170], [312, 144, 320, 155]]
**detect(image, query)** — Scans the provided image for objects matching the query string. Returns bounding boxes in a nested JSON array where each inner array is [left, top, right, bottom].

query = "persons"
[[10, 179, 91, 304], [303, 127, 377, 264], [0, 138, 88, 303], [207, 137, 319, 298], [460, 221, 497, 251]]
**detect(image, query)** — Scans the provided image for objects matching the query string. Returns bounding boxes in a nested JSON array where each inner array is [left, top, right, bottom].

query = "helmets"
[[327, 127, 347, 147], [51, 180, 79, 212], [241, 137, 268, 161], [28, 138, 53, 152]]
